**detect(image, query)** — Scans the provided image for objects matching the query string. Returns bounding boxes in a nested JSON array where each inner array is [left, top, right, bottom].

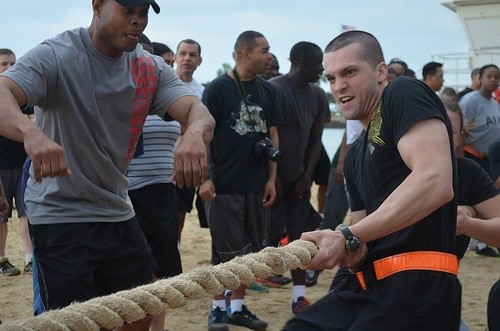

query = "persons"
[[0, 49, 35, 276], [458, 65, 500, 331], [0, 0, 216, 331], [386, 58, 458, 101], [199, 31, 286, 331], [330, 95, 500, 331], [246, 41, 364, 315], [281, 31, 462, 331], [126, 34, 206, 331]]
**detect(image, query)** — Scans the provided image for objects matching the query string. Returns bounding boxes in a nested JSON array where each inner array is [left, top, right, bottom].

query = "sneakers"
[[208, 305, 229, 331], [256, 274, 292, 287], [306, 268, 319, 287], [22, 259, 33, 274], [291, 297, 310, 315], [245, 282, 268, 294], [0, 257, 21, 277], [227, 305, 268, 331]]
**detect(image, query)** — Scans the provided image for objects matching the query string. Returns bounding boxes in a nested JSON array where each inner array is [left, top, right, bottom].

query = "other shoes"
[[475, 245, 500, 257]]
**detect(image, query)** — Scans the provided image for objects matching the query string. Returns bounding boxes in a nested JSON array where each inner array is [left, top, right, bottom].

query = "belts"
[[356, 251, 458, 290]]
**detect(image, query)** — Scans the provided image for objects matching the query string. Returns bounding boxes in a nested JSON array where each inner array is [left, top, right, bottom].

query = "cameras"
[[252, 137, 284, 163]]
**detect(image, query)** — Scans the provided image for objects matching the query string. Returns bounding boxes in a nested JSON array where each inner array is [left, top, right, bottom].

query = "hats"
[[114, 0, 160, 15]]
